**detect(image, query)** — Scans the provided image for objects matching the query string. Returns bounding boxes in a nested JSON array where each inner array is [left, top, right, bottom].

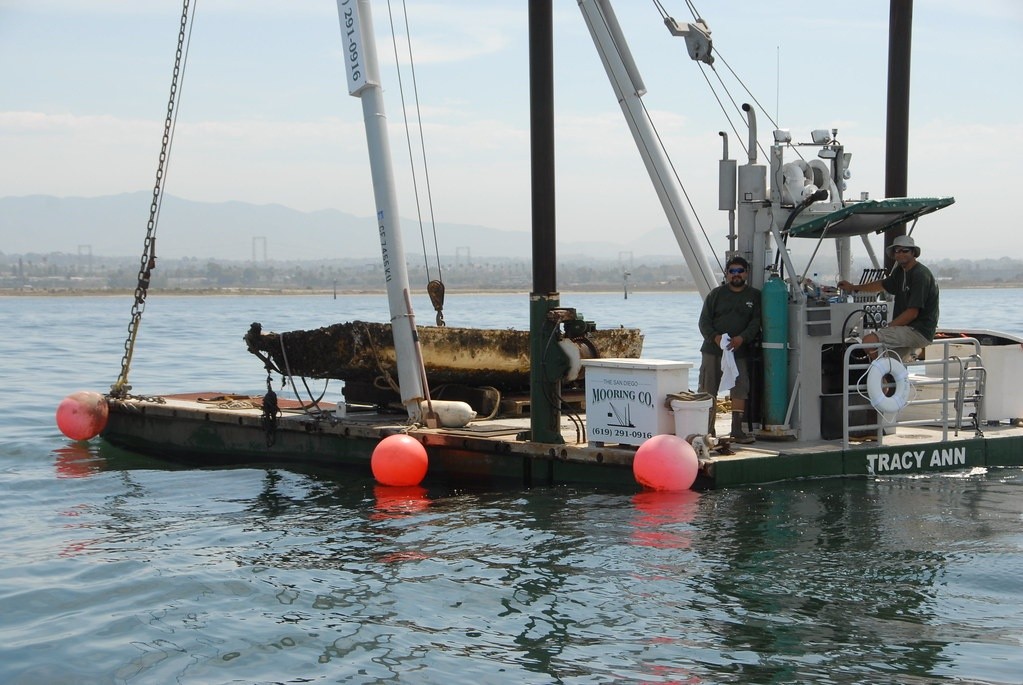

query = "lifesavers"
[[866, 356, 912, 414]]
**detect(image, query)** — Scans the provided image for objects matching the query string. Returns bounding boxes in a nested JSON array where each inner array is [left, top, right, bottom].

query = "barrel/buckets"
[[670, 398, 713, 440]]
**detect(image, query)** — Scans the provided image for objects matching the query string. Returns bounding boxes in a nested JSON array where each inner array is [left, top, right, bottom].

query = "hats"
[[886, 235, 921, 258]]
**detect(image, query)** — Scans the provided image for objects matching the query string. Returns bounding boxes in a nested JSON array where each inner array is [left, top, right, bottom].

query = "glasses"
[[894, 247, 910, 253], [729, 268, 745, 274]]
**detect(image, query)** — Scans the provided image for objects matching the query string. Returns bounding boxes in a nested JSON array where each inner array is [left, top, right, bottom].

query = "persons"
[[697, 258, 762, 443], [837, 234, 939, 442]]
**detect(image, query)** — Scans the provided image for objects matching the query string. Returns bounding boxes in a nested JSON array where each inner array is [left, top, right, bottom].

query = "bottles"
[[813, 273, 820, 299]]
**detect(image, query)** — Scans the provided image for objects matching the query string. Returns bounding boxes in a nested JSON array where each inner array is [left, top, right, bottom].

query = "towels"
[[717, 332, 739, 393]]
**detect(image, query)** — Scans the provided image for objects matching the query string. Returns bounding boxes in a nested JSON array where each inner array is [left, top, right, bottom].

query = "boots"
[[729, 410, 755, 443]]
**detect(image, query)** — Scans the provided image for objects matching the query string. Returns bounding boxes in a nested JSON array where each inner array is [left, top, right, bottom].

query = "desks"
[[577, 357, 695, 448]]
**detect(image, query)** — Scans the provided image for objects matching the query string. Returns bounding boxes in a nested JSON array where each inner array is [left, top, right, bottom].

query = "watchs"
[[884, 323, 888, 327]]
